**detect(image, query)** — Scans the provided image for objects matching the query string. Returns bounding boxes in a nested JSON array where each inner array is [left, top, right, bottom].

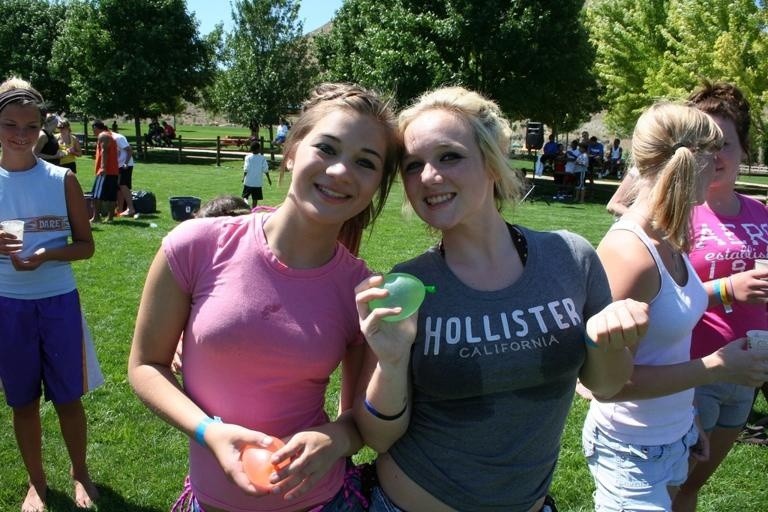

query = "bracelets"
[[711, 276, 736, 315], [193, 415, 222, 448], [360, 396, 408, 422]]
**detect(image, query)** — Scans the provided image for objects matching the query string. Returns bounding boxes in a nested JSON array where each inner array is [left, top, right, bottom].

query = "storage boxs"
[[83, 194, 93, 218]]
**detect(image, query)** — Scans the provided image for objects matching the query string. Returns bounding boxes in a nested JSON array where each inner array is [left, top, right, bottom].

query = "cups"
[[755, 259, 768, 300], [746, 329, 768, 355], [0, 220, 25, 252]]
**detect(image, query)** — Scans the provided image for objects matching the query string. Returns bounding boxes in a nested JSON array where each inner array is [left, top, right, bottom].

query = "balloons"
[[368, 272, 435, 322]]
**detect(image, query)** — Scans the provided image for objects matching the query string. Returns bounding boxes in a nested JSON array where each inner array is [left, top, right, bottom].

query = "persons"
[[112, 121, 117, 132], [242, 141, 271, 208], [351, 88, 650, 512], [537, 129, 627, 203], [32, 114, 135, 222], [129, 82, 406, 512], [272, 117, 290, 151], [249, 115, 261, 139], [171, 197, 251, 380], [574, 103, 767, 512], [669, 79, 768, 512], [519, 168, 534, 201], [145, 116, 176, 148], [0, 77, 107, 512]]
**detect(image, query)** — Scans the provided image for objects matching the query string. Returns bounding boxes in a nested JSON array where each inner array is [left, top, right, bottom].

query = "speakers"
[[526, 121, 544, 150]]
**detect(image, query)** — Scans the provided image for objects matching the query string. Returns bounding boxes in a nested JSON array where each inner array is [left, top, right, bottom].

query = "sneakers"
[[735, 425, 768, 446]]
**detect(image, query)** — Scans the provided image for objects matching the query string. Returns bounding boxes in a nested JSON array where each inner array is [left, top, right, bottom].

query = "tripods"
[[516, 150, 551, 206]]
